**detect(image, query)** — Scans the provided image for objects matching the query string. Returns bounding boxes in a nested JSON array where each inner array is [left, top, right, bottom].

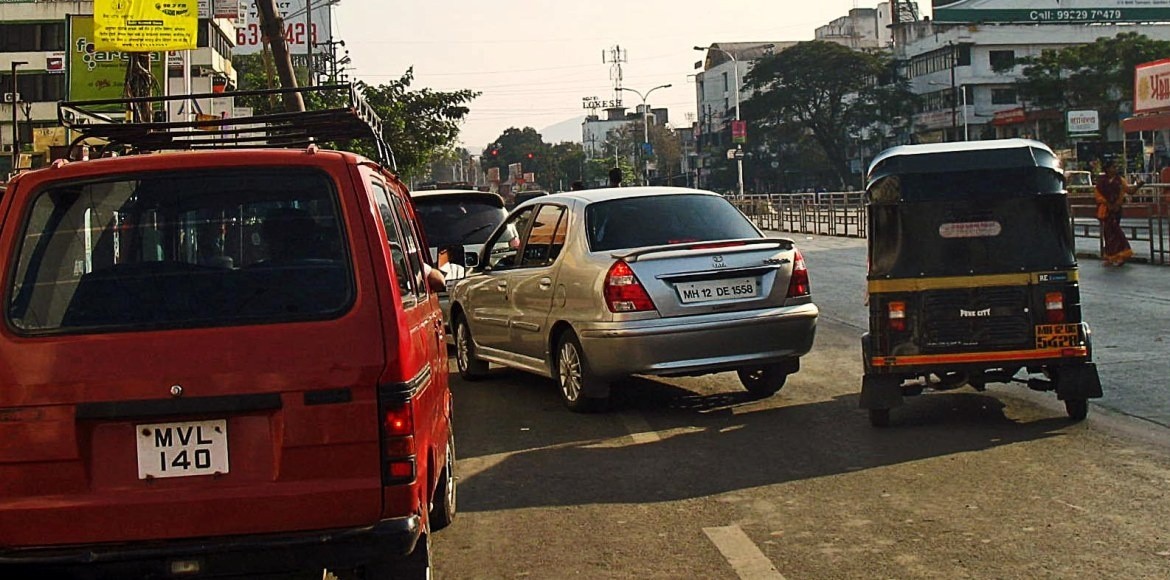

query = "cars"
[[405, 189, 818, 412]]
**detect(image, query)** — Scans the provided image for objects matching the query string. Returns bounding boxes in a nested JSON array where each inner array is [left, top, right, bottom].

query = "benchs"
[[58, 254, 352, 330]]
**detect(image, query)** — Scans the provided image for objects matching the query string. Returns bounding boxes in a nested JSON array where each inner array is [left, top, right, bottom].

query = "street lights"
[[614, 84, 673, 186], [928, 79, 968, 141], [693, 43, 777, 202]]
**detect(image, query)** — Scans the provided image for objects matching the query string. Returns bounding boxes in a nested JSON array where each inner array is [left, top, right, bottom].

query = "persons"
[[1095, 161, 1146, 266], [439, 248, 464, 279], [423, 261, 445, 292]]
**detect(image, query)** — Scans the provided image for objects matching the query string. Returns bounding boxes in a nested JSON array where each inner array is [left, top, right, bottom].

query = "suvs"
[[1, 83, 455, 580]]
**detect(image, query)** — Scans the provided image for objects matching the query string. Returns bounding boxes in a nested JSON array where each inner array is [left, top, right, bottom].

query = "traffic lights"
[[526, 152, 534, 159], [489, 149, 499, 158]]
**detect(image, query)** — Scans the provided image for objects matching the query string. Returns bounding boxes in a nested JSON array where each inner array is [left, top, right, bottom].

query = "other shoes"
[[1116, 254, 1123, 267], [1104, 257, 1110, 267]]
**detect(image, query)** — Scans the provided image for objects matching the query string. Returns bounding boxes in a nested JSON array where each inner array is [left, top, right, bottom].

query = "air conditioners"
[[4, 93, 21, 103]]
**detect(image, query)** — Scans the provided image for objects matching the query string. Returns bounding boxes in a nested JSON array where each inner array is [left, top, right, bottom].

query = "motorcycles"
[[860, 138, 1104, 430]]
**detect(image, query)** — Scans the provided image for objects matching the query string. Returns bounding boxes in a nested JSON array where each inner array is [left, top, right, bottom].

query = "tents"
[[1122, 114, 1170, 176]]
